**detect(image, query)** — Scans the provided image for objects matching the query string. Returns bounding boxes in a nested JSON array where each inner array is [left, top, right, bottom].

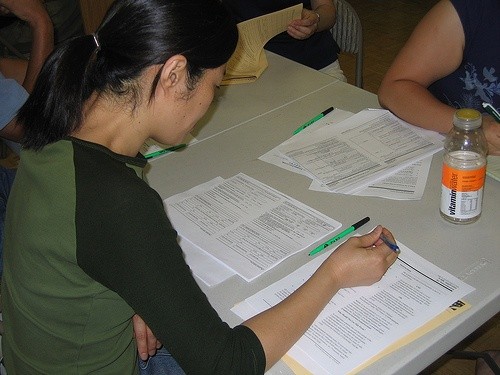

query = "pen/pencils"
[[145, 144, 188, 159], [307, 216, 371, 256], [294, 106, 334, 135], [378, 233, 400, 253], [482, 102, 500, 121]]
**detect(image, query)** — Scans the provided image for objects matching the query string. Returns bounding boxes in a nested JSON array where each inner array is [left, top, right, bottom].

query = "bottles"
[[440, 108, 488, 224]]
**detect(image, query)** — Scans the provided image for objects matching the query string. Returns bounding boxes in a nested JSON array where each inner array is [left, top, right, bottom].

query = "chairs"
[[332, 0, 364, 88]]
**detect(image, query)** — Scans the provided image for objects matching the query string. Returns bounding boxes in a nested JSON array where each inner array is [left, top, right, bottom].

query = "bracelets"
[[314, 11, 321, 33]]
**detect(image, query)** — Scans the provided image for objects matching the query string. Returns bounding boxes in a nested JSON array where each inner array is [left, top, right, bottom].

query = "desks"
[[139, 49, 500, 375]]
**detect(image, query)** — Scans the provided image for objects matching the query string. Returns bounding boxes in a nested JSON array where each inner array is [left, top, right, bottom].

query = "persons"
[[0, 0, 55, 269], [222, 0, 349, 83], [0, 0, 401, 375], [378, 0, 500, 156]]
